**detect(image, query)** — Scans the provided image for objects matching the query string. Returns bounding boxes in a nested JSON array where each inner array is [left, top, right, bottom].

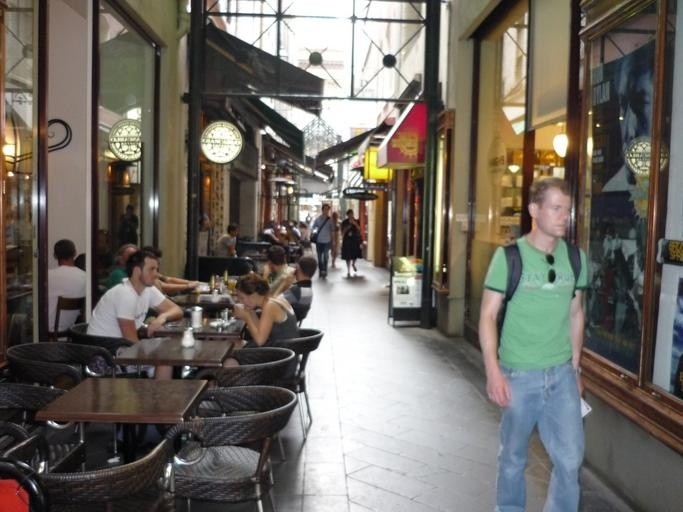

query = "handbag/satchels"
[[310, 230, 317, 242]]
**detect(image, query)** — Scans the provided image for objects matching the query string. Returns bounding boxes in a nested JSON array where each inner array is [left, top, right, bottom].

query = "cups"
[[233, 303, 244, 311], [184, 306, 203, 329]]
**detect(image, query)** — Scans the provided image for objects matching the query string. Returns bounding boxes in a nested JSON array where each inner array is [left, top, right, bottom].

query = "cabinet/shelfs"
[[498, 165, 552, 241]]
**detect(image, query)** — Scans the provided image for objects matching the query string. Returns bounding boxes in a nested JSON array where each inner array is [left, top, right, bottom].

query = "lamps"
[[199, 120, 244, 166], [553, 120, 568, 159], [624, 136, 667, 178], [107, 118, 141, 162], [507, 164, 521, 175]]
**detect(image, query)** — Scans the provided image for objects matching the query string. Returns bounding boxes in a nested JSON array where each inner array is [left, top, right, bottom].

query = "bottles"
[[180, 327, 195, 348]]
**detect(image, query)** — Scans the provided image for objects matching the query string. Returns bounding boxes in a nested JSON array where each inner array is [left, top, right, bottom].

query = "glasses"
[[546, 253, 555, 284]]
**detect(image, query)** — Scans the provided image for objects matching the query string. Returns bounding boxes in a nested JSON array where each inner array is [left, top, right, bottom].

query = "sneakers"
[[322, 273, 327, 280], [347, 273, 350, 278], [351, 263, 356, 271]]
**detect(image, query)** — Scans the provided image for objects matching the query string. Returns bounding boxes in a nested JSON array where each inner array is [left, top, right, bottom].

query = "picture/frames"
[[648, 258, 682, 401]]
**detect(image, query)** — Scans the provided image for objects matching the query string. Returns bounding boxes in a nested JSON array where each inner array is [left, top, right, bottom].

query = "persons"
[[598, 224, 625, 264], [308, 202, 333, 282], [94, 203, 139, 282], [84, 249, 186, 378], [599, 41, 655, 191], [339, 204, 362, 279], [46, 238, 83, 335], [142, 245, 200, 295], [475, 176, 594, 512], [274, 258, 316, 325], [229, 274, 301, 347], [190, 194, 384, 294], [71, 251, 87, 271]]
